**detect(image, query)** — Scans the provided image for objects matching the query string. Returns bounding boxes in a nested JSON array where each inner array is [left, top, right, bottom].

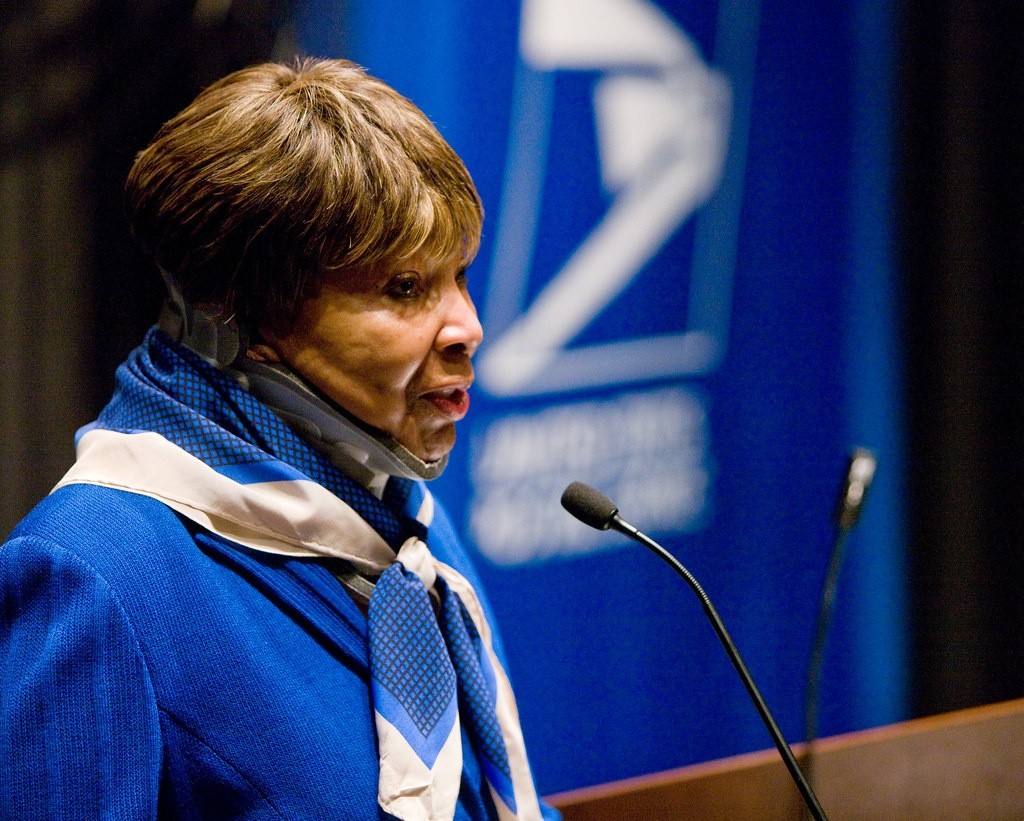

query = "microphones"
[[562, 479, 827, 821], [796, 444, 880, 820]]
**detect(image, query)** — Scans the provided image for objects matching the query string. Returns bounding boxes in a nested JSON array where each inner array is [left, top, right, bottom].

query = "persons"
[[0, 58, 562, 820]]
